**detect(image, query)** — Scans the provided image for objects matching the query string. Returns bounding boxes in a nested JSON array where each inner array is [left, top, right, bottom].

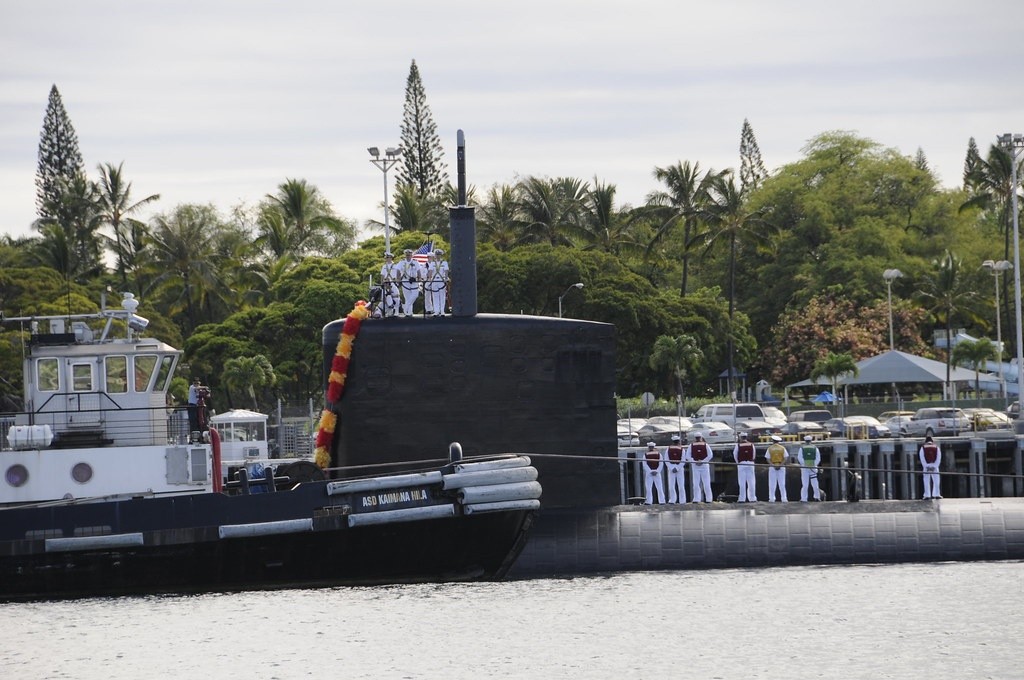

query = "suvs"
[[899, 408, 972, 437]]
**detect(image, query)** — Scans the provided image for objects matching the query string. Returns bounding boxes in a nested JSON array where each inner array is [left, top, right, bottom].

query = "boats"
[[1, 291, 543, 602]]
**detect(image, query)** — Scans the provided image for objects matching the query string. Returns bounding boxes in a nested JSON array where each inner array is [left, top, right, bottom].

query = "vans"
[[689, 404, 766, 427]]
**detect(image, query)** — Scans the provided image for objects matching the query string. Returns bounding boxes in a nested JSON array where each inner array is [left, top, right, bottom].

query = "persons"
[[686, 432, 713, 504], [187, 378, 210, 432], [798, 435, 821, 502], [664, 435, 687, 504], [420, 249, 450, 317], [396, 248, 422, 317], [764, 435, 789, 502], [919, 435, 943, 500], [732, 432, 756, 503], [642, 441, 666, 505], [380, 251, 401, 315]]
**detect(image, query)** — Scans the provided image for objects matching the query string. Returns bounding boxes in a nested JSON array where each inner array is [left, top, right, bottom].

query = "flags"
[[412, 241, 432, 264]]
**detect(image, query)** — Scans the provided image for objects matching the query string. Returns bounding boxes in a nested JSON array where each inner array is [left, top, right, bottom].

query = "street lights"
[[369, 146, 404, 253], [982, 260, 1013, 380], [882, 268, 903, 401], [558, 282, 583, 317]]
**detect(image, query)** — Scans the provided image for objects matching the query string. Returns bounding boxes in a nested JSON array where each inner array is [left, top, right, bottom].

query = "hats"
[[647, 442, 656, 447], [403, 249, 413, 254], [671, 435, 680, 441], [434, 249, 444, 255], [771, 436, 782, 442], [427, 251, 434, 257], [739, 432, 748, 437], [925, 436, 933, 442], [803, 436, 812, 440], [384, 252, 394, 258], [694, 432, 702, 438]]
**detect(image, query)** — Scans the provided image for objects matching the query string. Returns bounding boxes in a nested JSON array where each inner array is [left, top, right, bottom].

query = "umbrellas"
[[813, 391, 842, 405]]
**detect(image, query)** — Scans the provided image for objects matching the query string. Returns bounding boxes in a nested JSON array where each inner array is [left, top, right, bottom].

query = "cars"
[[617, 402, 1020, 446]]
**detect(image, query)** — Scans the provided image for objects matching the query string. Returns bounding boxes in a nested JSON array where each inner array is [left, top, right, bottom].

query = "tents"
[[785, 349, 1007, 401]]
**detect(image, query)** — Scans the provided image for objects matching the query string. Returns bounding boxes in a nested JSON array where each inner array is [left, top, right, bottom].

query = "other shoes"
[[935, 496, 943, 499], [924, 497, 932, 500], [425, 311, 433, 314], [441, 315, 445, 317], [433, 315, 439, 317], [800, 501, 807, 503], [815, 499, 821, 502]]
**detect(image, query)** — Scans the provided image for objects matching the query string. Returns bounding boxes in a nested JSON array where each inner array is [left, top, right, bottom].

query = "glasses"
[[194, 382, 200, 384], [385, 256, 390, 258]]
